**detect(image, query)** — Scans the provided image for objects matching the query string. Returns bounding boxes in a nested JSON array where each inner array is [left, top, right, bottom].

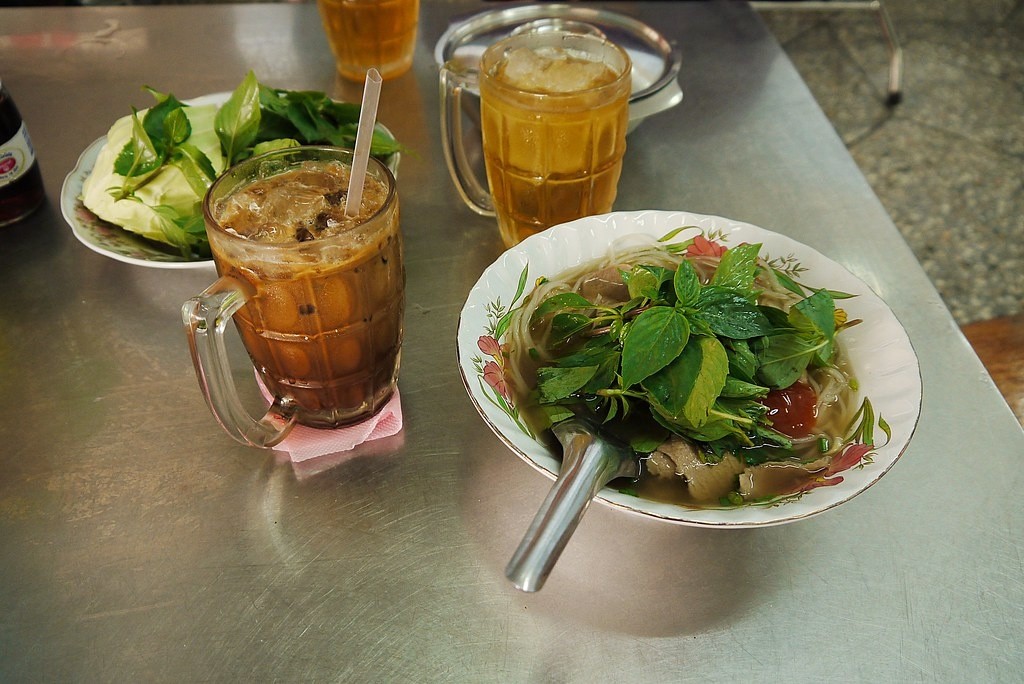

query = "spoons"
[[505, 420, 642, 594]]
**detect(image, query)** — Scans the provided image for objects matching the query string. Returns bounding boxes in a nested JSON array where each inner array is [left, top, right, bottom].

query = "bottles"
[[0, 83, 44, 220]]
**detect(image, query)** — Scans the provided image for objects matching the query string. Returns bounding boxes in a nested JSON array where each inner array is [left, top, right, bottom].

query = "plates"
[[60, 92, 400, 268]]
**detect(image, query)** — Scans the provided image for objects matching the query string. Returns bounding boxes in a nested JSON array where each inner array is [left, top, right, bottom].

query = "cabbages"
[[81, 101, 225, 248]]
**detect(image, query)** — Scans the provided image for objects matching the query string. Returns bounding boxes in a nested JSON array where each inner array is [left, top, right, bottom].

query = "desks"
[[0, 0, 1024, 684]]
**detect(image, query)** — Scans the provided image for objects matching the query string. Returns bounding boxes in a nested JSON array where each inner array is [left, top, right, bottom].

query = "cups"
[[316, 0, 419, 81], [181, 148, 405, 447], [443, 21, 632, 251]]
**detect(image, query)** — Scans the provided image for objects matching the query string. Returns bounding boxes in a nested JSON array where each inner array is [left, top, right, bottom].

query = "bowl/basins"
[[455, 210, 924, 530], [433, 2, 683, 137]]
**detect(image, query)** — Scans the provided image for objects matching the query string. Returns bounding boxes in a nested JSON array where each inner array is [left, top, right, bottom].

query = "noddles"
[[505, 231, 860, 505]]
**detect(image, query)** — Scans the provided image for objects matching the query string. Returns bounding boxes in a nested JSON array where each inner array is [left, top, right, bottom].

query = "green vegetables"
[[519, 241, 860, 502], [106, 67, 419, 258]]
